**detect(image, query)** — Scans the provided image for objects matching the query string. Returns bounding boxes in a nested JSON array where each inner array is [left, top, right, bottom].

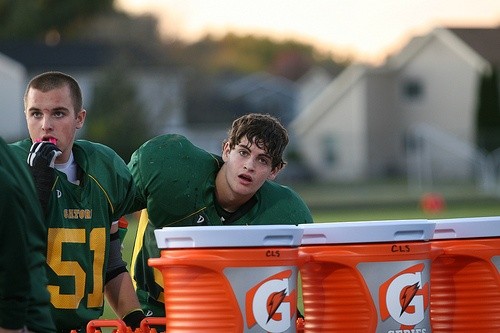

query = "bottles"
[[118, 216, 128, 245]]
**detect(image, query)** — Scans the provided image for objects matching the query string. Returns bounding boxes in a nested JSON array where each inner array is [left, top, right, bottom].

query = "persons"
[[0, 134, 58, 333], [9, 72, 146, 333], [126, 113, 314, 333]]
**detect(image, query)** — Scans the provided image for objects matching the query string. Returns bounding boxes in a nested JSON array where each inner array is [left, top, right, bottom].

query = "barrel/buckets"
[[429, 216, 500, 333], [296, 219, 437, 333], [148, 224, 304, 333]]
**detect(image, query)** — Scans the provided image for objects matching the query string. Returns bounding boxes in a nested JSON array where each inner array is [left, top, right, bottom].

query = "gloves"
[[26, 140, 63, 198], [122, 309, 145, 332]]
